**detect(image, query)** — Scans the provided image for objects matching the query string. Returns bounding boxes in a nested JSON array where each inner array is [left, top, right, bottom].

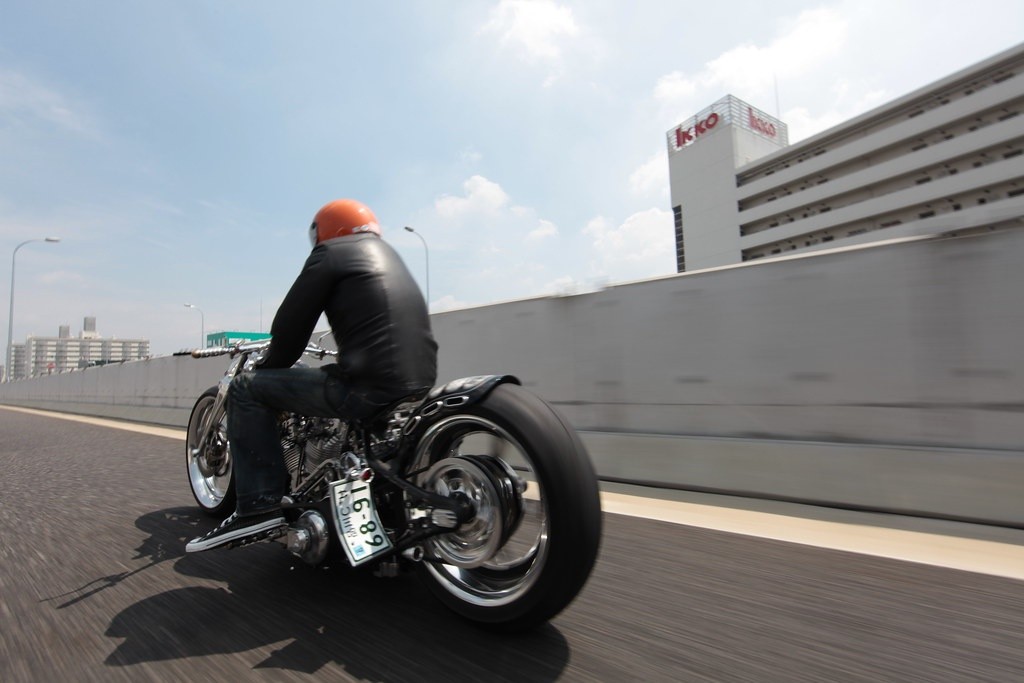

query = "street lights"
[[404, 225, 430, 312], [5, 236, 61, 382], [183, 303, 204, 350]]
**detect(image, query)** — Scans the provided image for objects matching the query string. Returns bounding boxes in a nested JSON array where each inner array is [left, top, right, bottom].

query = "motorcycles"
[[181, 339, 602, 640]]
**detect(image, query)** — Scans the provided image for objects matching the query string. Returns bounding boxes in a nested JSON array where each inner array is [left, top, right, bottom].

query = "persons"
[[187, 200, 440, 553]]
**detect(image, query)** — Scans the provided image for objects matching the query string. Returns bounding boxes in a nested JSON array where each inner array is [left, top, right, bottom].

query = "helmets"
[[309, 198, 380, 250]]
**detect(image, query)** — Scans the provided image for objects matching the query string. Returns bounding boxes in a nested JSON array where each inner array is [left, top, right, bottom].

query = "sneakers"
[[185, 510, 286, 552]]
